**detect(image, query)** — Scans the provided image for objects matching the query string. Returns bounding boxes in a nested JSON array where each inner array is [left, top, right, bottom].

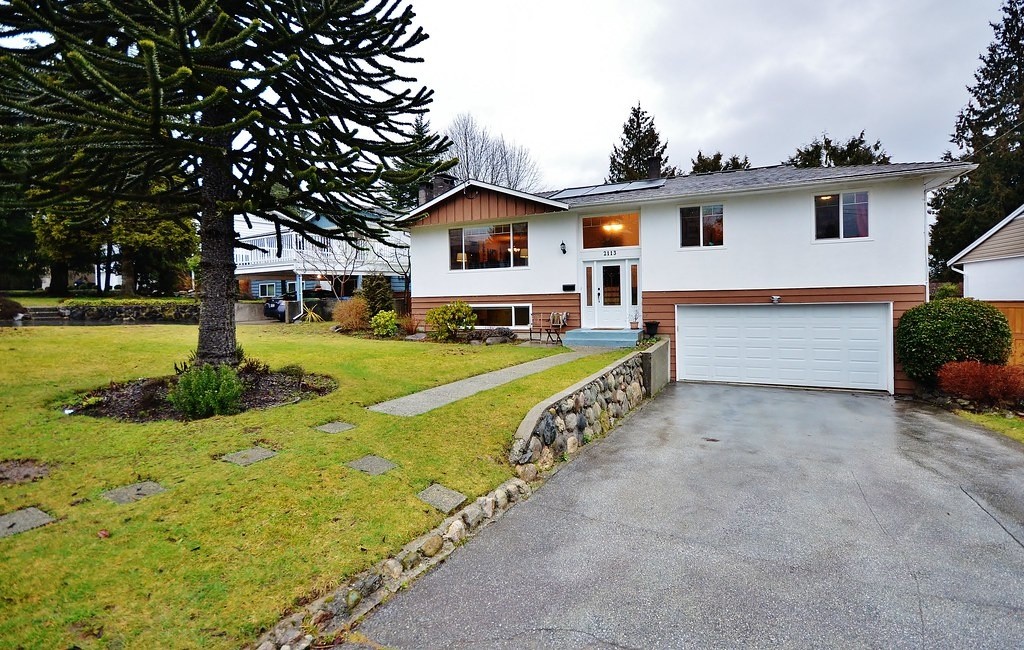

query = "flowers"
[[647, 320, 658, 322]]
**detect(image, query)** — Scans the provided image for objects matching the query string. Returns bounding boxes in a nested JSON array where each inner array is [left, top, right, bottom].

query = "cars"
[[264, 288, 349, 323], [74, 279, 95, 290]]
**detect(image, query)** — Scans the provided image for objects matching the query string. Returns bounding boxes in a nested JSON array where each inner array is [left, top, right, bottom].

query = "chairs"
[[545, 311, 570, 345]]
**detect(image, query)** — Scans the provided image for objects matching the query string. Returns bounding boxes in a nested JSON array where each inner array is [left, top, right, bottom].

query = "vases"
[[644, 322, 660, 338]]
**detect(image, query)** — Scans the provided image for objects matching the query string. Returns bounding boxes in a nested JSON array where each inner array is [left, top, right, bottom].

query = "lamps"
[[456, 253, 467, 269], [560, 240, 566, 254], [520, 248, 528, 266]]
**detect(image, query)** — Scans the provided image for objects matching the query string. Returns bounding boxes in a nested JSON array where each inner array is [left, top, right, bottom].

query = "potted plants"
[[628, 310, 641, 328]]
